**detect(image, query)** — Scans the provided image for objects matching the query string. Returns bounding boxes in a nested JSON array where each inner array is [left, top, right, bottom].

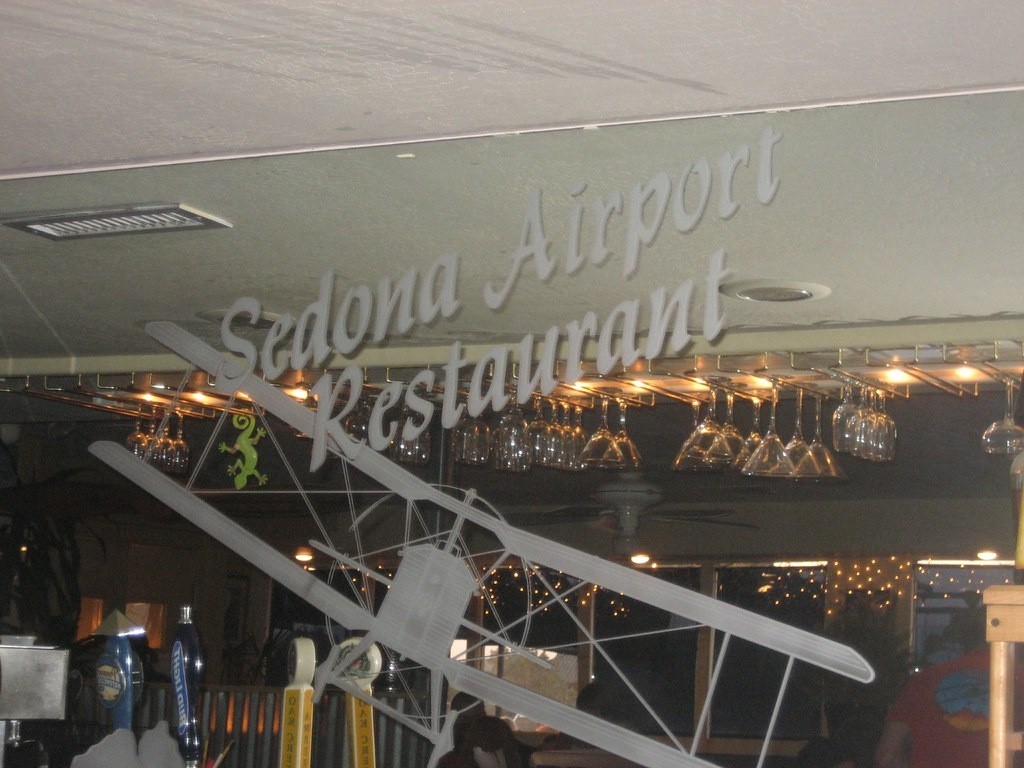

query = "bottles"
[[167, 604, 204, 765]]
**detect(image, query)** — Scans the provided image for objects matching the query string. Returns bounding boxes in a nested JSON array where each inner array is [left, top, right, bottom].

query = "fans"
[[503, 468, 760, 535]]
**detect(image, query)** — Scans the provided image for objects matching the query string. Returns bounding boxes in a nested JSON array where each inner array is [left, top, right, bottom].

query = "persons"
[[873, 612, 1024, 768], [435, 693, 533, 768], [127, 635, 172, 683], [539, 683, 631, 750], [801, 699, 874, 768]]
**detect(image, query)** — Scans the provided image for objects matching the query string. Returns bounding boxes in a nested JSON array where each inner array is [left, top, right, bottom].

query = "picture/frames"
[[222, 572, 252, 648]]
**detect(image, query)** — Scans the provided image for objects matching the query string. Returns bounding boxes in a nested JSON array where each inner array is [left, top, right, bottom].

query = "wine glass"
[[126, 404, 189, 474], [981, 371, 1024, 490], [290, 371, 897, 481]]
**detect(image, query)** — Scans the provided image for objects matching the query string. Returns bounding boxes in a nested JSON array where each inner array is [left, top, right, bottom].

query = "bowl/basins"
[[0, 635, 37, 647]]
